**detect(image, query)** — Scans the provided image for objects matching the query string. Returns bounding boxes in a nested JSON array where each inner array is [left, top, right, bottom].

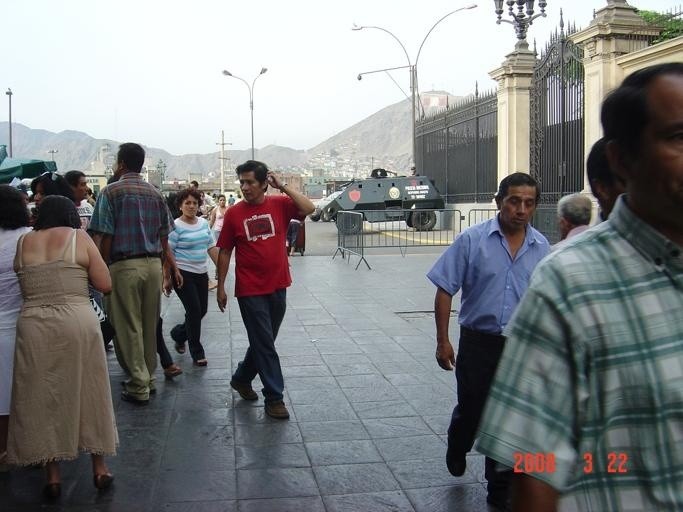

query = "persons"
[[549, 194, 595, 251], [587, 136, 624, 225], [0, 142, 316, 495], [426, 173, 553, 477], [471, 58, 683, 512]]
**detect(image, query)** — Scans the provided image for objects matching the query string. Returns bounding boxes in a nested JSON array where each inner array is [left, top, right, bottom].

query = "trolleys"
[[286, 222, 305, 257]]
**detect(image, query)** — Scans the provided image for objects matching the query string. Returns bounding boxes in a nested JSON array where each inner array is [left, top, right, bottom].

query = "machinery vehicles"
[[323, 172, 443, 233]]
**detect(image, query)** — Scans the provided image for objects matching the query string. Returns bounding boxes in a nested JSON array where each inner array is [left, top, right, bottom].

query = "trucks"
[[302, 176, 364, 203]]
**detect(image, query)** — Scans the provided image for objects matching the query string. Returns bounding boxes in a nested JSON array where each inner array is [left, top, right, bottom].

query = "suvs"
[[307, 190, 341, 223]]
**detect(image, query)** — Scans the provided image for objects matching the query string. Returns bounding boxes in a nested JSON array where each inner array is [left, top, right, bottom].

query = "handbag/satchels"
[[87, 286, 108, 322]]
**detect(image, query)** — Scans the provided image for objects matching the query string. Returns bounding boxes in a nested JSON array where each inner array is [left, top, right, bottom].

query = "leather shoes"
[[93, 474, 114, 489], [445, 434, 467, 476], [486, 486, 510, 510], [44, 482, 62, 497]]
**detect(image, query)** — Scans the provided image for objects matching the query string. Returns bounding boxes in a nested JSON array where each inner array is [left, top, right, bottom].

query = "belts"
[[122, 254, 160, 261]]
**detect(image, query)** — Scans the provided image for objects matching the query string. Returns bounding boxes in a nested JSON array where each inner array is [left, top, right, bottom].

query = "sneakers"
[[164, 365, 183, 380], [194, 358, 208, 366], [120, 379, 156, 393], [174, 340, 186, 353], [230, 379, 259, 401], [120, 389, 149, 406], [265, 399, 290, 419]]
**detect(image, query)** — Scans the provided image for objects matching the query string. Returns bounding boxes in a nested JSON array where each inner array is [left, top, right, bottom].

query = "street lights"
[[102, 166, 112, 184], [350, 3, 478, 176], [221, 66, 268, 164], [5, 87, 13, 156], [48, 149, 58, 161], [154, 158, 168, 192], [493, 0, 547, 52]]
